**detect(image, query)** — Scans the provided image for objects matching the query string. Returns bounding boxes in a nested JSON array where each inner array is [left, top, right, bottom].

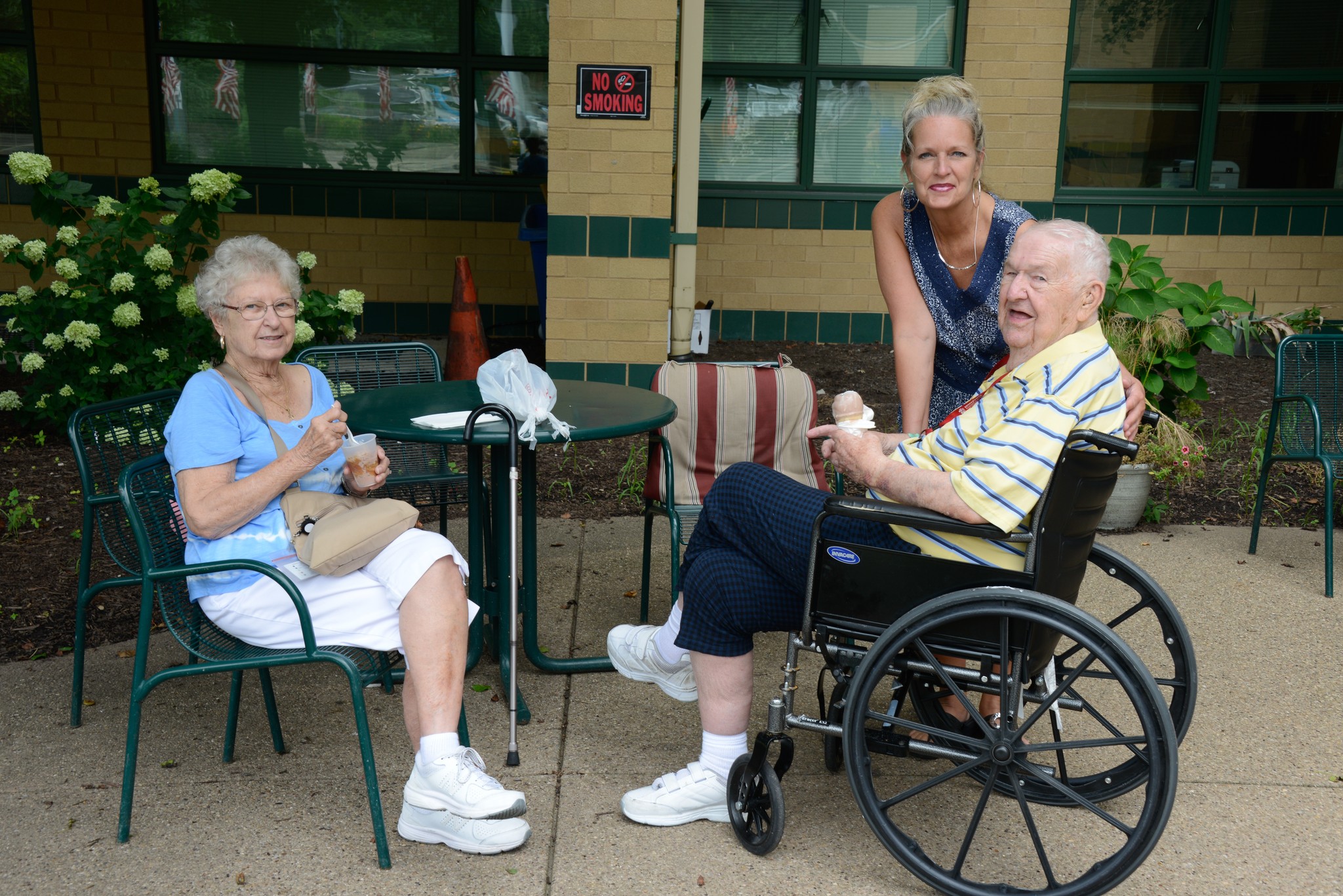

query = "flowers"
[[1100, 317, 1208, 469]]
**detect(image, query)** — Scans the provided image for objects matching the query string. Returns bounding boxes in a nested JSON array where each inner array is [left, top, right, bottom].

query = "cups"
[[341, 433, 379, 487]]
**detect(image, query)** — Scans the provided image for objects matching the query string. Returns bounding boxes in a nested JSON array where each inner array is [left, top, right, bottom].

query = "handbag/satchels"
[[476, 348, 571, 443], [280, 491, 420, 577]]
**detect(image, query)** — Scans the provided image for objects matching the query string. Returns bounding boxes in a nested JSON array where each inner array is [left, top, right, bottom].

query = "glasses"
[[217, 298, 299, 320]]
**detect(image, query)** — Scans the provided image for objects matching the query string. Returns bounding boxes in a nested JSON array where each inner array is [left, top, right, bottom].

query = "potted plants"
[[1211, 319, 1310, 357]]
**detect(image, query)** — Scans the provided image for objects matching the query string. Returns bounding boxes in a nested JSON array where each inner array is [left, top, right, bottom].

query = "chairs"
[[640, 353, 841, 625], [64, 342, 490, 871], [1248, 334, 1343, 598]]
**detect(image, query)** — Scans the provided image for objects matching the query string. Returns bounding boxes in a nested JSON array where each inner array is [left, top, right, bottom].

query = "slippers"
[[907, 712, 974, 760], [980, 713, 1027, 759]]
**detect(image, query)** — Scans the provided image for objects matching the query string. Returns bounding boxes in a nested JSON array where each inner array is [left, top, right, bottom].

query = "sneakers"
[[397, 798, 532, 855], [607, 624, 698, 703], [403, 745, 526, 820], [620, 761, 755, 827]]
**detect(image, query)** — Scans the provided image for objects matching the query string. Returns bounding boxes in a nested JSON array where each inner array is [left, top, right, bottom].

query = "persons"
[[607, 218, 1128, 827], [163, 234, 533, 855], [872, 75, 1146, 760]]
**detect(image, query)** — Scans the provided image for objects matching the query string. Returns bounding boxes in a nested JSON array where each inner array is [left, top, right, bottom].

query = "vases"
[[1099, 463, 1152, 531]]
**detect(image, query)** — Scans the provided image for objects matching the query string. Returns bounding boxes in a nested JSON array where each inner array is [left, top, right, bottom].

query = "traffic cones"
[[440, 254, 493, 381]]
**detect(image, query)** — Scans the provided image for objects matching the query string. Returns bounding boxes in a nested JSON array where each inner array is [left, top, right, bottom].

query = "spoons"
[[331, 405, 360, 446]]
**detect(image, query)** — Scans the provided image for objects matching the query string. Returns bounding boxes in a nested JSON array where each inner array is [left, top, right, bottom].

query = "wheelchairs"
[[724, 401, 1199, 896]]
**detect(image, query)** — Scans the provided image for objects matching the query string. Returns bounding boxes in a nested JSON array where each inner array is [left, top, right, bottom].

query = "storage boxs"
[[668, 302, 711, 353]]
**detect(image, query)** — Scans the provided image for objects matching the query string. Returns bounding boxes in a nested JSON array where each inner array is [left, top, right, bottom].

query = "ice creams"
[[832, 390, 865, 437]]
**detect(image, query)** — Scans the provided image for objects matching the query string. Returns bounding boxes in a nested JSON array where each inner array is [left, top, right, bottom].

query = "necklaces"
[[224, 358, 295, 420], [930, 190, 979, 270]]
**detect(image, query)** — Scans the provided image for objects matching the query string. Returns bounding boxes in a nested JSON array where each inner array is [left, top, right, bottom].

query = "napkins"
[[408, 411, 504, 429]]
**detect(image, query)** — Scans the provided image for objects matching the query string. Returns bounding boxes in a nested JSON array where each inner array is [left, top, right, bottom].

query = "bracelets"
[[341, 466, 372, 498]]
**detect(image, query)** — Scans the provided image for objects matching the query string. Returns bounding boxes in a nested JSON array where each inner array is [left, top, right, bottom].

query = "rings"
[[389, 470, 392, 474]]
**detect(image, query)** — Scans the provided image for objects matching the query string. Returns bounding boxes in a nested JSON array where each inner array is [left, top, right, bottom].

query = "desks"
[[339, 379, 680, 724]]
